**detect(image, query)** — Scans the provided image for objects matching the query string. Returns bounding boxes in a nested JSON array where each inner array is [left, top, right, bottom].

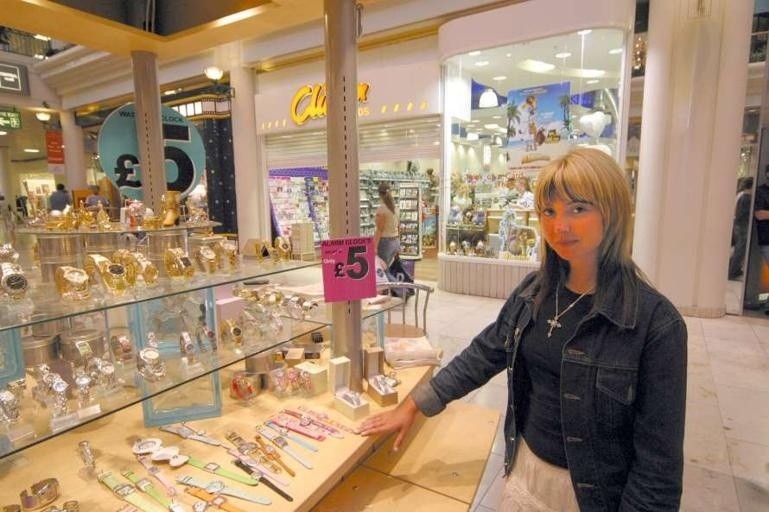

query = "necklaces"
[[545, 276, 598, 340]]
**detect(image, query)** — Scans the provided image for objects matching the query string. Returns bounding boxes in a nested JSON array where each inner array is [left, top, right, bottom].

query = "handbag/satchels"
[[389, 259, 415, 298]]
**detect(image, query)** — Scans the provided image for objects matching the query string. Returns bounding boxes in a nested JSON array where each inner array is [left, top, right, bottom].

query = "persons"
[[45, 183, 71, 212], [16, 196, 29, 217], [372, 183, 402, 270], [451, 183, 473, 213], [727, 177, 755, 281], [525, 93, 539, 131], [744, 165, 768, 311], [83, 185, 110, 212], [507, 176, 535, 209], [347, 147, 688, 509], [501, 174, 517, 195], [424, 168, 439, 238]]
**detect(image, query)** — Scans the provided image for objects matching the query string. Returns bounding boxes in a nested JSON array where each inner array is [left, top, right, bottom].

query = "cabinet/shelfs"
[[447, 169, 543, 263], [267, 166, 440, 264]]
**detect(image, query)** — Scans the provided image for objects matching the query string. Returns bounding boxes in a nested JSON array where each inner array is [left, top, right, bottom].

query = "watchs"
[[1, 234, 400, 510]]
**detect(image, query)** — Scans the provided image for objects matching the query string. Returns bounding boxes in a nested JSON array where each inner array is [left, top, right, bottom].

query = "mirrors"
[[727, 105, 763, 316]]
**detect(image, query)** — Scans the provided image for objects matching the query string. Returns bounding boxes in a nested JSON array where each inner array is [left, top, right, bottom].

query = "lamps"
[[202, 67, 235, 97], [35, 112, 61, 130]]
